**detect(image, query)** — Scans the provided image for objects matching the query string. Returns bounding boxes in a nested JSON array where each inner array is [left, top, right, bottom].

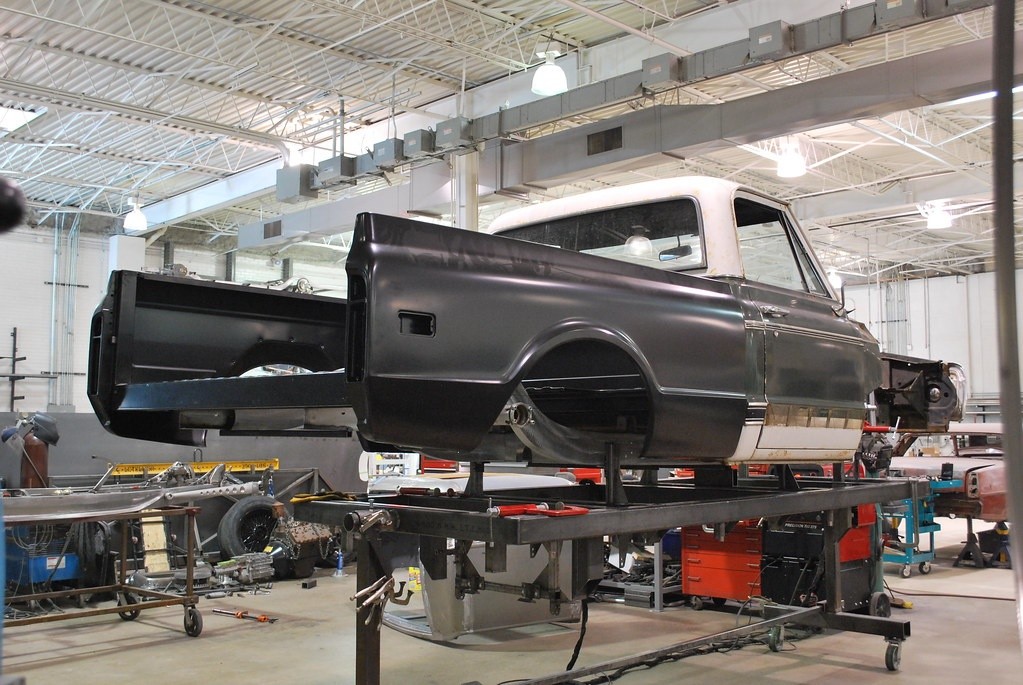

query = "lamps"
[[123, 194, 147, 231], [531, 42, 569, 95]]
